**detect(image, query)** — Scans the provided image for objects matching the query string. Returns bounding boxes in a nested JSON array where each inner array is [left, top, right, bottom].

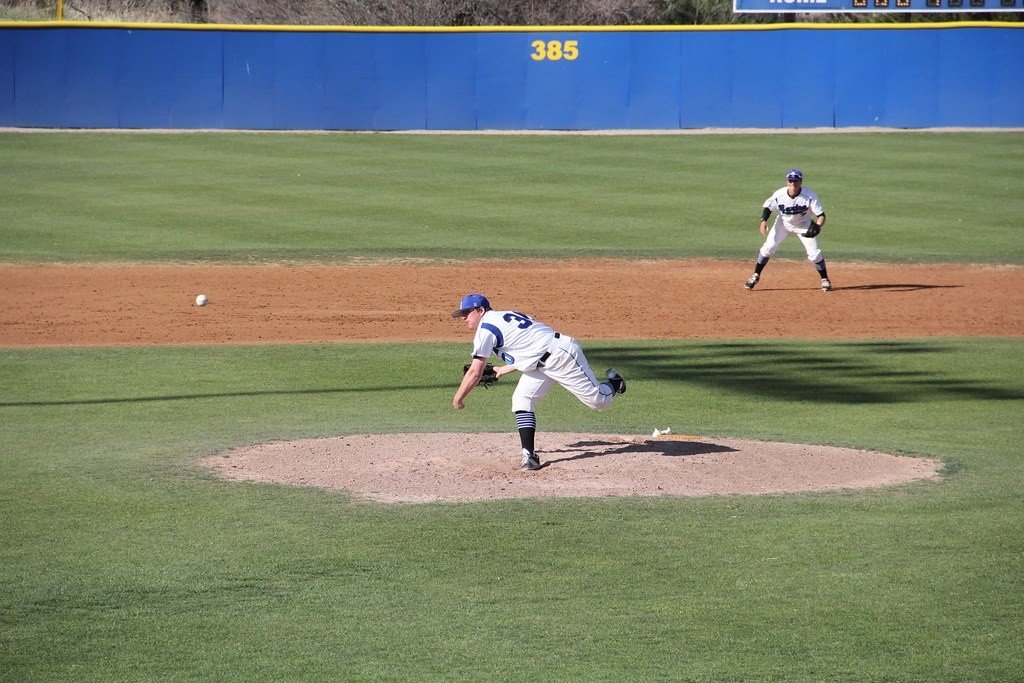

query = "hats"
[[452, 294, 492, 319], [786, 171, 803, 182]]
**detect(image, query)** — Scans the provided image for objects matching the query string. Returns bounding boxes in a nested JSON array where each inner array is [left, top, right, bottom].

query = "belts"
[[535, 332, 563, 370]]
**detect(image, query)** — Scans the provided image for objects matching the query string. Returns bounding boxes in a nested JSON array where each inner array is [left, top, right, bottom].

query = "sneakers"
[[744, 276, 762, 289], [523, 454, 541, 471], [821, 279, 834, 290], [605, 368, 627, 394]]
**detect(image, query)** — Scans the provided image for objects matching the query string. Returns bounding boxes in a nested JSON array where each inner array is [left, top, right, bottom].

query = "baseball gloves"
[[463, 362, 498, 383], [801, 221, 820, 238]]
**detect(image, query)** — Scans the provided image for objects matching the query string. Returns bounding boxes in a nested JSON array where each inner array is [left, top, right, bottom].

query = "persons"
[[744, 169, 832, 293], [452, 294, 626, 471]]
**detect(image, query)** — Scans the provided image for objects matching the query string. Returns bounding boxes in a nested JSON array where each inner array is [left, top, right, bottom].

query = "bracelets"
[[761, 220, 765, 223]]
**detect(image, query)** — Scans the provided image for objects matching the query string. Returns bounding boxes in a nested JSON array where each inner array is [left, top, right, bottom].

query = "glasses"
[[789, 181, 799, 184]]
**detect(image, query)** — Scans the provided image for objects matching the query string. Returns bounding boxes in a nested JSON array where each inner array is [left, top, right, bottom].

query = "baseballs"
[[196, 295, 207, 306]]
